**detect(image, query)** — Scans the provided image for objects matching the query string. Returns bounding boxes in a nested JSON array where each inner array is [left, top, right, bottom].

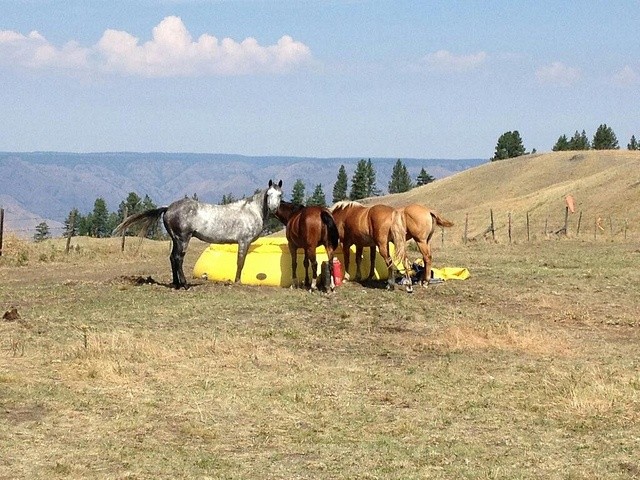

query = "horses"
[[111, 178, 283, 290], [329, 200, 413, 292], [274, 199, 339, 294], [366, 203, 456, 289]]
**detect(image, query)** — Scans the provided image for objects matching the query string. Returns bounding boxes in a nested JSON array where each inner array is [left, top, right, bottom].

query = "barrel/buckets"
[[322, 257, 343, 291]]
